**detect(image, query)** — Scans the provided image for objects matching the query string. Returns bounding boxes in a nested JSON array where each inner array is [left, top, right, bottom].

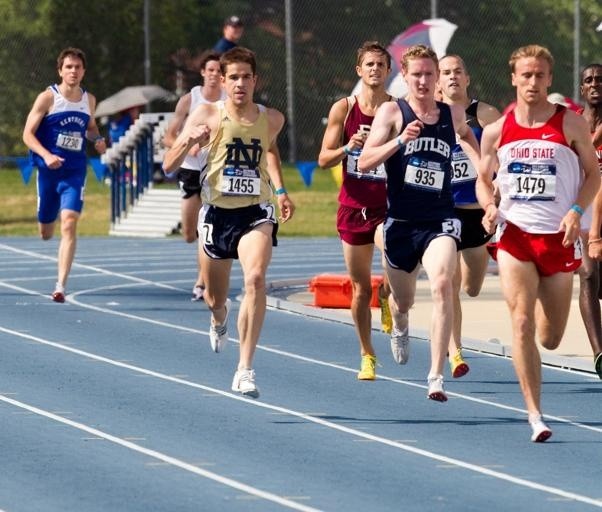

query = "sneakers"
[[231, 370, 261, 398], [379, 283, 393, 335], [51, 290, 66, 303], [528, 412, 552, 444], [357, 354, 378, 381], [207, 297, 234, 354], [191, 285, 203, 303], [426, 372, 448, 402], [449, 352, 470, 380], [390, 325, 410, 365], [592, 352, 602, 381]]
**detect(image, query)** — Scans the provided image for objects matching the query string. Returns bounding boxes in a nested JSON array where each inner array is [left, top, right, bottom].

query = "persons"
[[588, 183, 602, 258], [22, 46, 106, 303], [434, 53, 501, 379], [213, 15, 243, 53], [318, 41, 400, 381], [162, 46, 296, 399], [102, 112, 132, 185], [571, 63, 602, 381], [162, 52, 227, 303], [358, 44, 482, 403], [474, 45, 600, 444]]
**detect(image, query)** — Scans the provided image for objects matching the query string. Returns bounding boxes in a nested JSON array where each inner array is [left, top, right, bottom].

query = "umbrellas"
[[94, 84, 172, 118], [350, 17, 458, 100]]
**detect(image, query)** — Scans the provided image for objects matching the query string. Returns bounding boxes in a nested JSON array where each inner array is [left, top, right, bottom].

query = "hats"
[[224, 15, 245, 28]]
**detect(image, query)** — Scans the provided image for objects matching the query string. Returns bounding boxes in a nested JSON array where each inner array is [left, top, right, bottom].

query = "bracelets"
[[571, 204, 583, 216], [344, 147, 350, 153], [587, 237, 602, 247], [397, 135, 404, 147], [93, 136, 106, 144], [275, 189, 287, 195]]
